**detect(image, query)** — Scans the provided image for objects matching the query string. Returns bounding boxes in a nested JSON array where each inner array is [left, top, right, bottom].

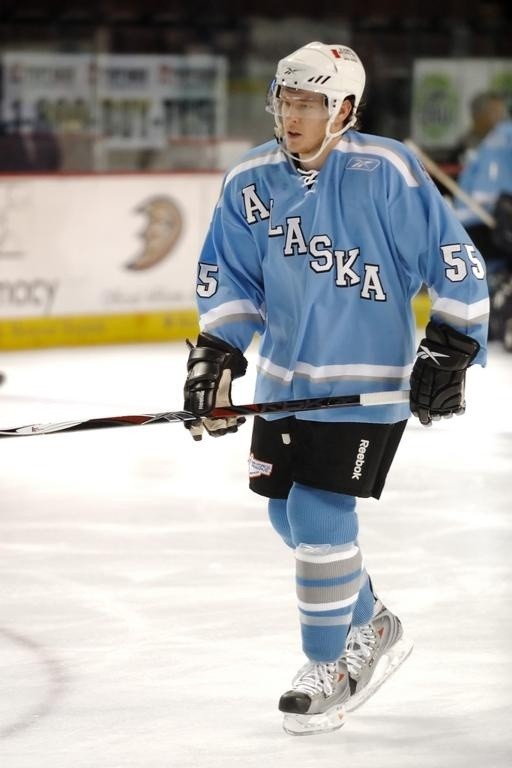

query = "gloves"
[[183, 332, 248, 439], [408, 321, 478, 426]]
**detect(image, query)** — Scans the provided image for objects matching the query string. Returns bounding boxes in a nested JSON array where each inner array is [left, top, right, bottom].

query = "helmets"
[[267, 40, 367, 139]]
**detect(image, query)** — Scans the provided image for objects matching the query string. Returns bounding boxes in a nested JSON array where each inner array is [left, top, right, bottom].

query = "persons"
[[183, 39, 492, 715], [452, 103, 512, 350], [448, 91, 509, 165], [0, 108, 62, 172]]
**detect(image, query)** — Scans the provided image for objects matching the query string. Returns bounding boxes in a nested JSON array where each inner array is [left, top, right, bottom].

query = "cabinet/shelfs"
[[0, 52, 229, 143]]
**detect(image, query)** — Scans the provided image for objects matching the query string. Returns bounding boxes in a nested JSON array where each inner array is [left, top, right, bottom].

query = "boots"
[[279, 622, 351, 714], [344, 573, 403, 695]]
